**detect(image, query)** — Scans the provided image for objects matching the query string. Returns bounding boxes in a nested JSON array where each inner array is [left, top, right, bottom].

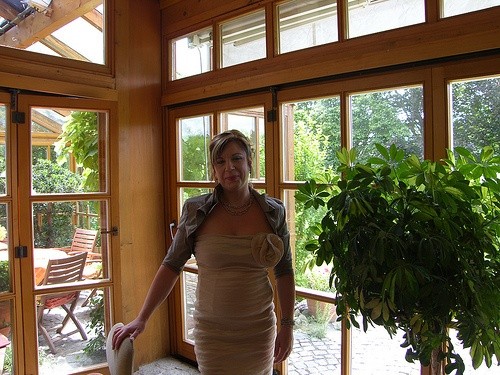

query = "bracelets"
[[281, 320, 295, 326]]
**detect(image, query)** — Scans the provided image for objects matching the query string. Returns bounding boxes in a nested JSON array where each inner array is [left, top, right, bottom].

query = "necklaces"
[[219, 197, 253, 215]]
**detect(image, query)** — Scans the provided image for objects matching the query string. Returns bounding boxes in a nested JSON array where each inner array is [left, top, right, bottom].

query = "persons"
[[112, 130, 295, 375]]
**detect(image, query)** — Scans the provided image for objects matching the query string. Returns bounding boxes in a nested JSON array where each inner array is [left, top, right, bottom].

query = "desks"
[[34, 247, 70, 286]]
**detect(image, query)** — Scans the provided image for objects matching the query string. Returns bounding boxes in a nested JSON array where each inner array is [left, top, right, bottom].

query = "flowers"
[[250, 231, 284, 269]]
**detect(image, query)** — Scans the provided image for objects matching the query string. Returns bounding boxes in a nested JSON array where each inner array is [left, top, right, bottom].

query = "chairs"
[[70, 228, 98, 268], [105, 320, 134, 375], [38, 251, 88, 355]]
